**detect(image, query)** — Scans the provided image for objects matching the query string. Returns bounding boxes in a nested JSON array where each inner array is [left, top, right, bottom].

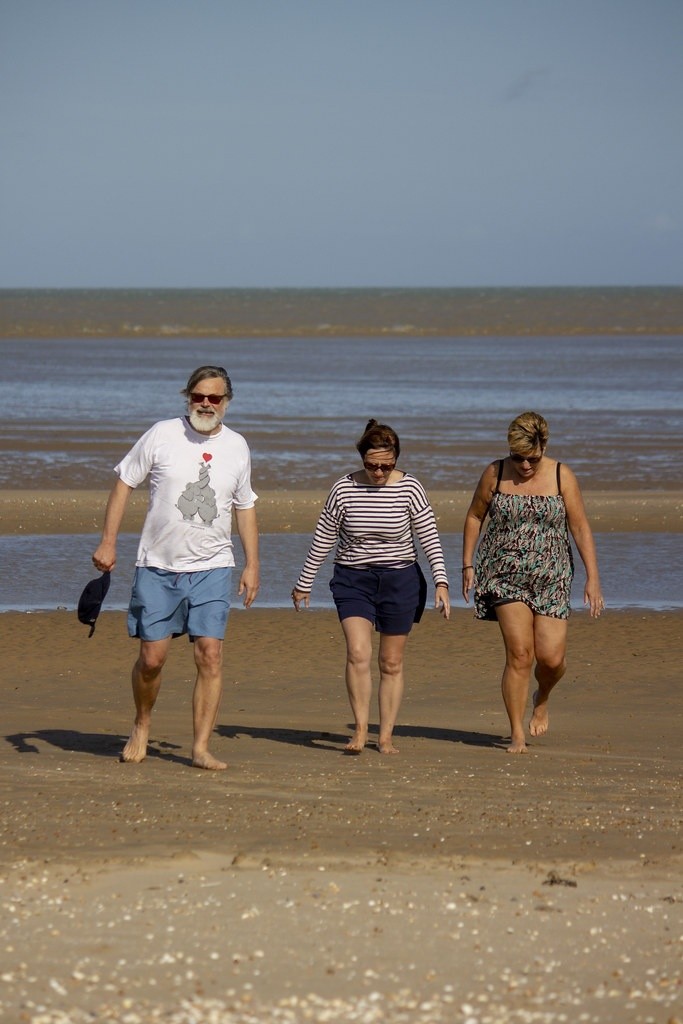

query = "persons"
[[92, 366, 260, 770], [291, 419, 451, 753], [462, 411, 605, 753]]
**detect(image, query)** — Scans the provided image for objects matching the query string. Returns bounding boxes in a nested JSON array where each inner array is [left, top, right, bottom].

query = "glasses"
[[190, 393, 227, 404], [510, 449, 543, 463], [362, 460, 397, 472]]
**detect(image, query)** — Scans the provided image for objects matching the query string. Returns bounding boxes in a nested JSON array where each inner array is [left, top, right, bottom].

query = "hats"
[[77, 571, 110, 638]]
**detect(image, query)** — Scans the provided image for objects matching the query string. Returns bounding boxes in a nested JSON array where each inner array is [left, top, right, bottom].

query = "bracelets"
[[438, 586, 447, 588], [462, 565, 473, 573]]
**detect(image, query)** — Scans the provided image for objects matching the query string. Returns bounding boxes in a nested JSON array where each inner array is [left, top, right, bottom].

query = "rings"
[[599, 601, 602, 603]]
